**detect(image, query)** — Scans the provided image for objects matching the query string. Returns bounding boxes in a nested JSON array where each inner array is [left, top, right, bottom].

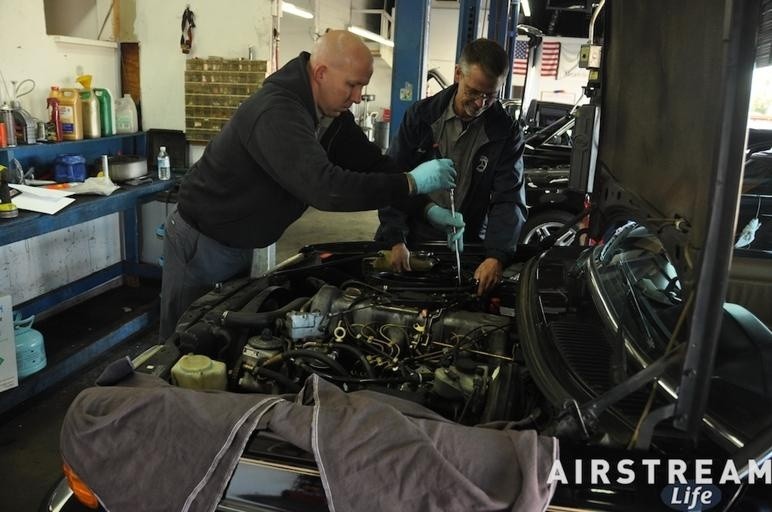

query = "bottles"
[[157, 147, 171, 180], [97, 155, 109, 177]]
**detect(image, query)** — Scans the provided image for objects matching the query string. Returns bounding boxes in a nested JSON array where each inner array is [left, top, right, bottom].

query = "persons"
[[159, 30, 466, 346], [374, 37, 529, 301]]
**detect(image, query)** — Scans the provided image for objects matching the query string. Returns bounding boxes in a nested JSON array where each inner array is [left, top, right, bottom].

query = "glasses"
[[460, 74, 503, 103]]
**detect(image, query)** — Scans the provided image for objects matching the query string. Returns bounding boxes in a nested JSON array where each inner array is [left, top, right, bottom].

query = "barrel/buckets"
[[93, 88, 112, 136], [79, 89, 102, 138], [114, 92, 139, 134], [50, 85, 84, 140]]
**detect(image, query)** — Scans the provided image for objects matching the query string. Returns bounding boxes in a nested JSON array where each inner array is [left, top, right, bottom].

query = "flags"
[[511, 40, 561, 80]]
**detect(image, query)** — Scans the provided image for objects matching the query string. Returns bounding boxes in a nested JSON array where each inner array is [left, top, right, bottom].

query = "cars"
[[736, 127, 771, 252], [515, 99, 579, 205]]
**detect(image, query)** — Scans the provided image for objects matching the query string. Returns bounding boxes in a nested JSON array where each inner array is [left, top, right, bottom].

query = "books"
[[7, 181, 76, 216]]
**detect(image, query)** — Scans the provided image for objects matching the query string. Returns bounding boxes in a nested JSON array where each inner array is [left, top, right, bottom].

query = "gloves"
[[426, 204, 467, 254], [409, 157, 460, 194]]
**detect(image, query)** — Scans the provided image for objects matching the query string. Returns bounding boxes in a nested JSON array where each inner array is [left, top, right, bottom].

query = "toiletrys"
[[45, 97, 64, 142]]
